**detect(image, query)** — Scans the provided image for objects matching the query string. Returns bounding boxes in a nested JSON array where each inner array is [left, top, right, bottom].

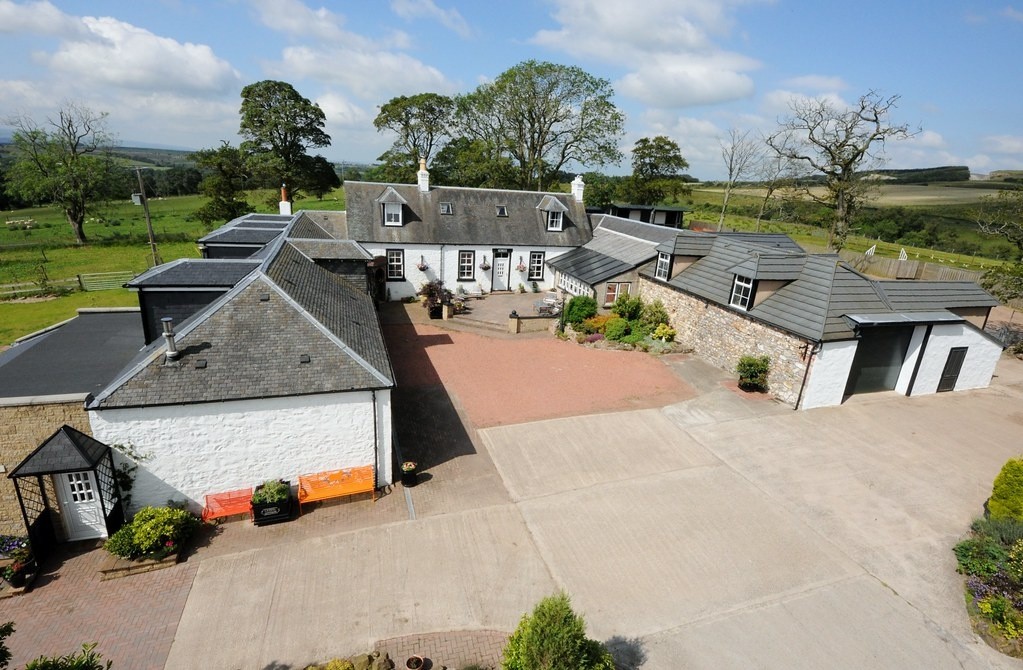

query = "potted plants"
[[532, 281, 538, 293], [736, 355, 772, 393], [14, 551, 35, 575], [249, 480, 298, 527], [415, 280, 443, 319], [518, 284, 525, 293]]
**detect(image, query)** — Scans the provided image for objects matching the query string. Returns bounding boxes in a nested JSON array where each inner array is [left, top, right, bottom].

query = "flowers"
[[479, 263, 490, 269], [4, 563, 20, 578], [515, 263, 526, 271], [416, 264, 428, 270], [402, 462, 416, 471]]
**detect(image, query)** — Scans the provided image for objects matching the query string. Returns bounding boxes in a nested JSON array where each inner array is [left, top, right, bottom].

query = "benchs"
[[202, 488, 253, 526], [298, 465, 376, 515]]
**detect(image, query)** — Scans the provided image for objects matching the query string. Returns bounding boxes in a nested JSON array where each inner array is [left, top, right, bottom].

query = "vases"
[[419, 269, 426, 271], [482, 268, 488, 270], [2, 566, 26, 588], [519, 269, 524, 272], [399, 462, 418, 487]]
